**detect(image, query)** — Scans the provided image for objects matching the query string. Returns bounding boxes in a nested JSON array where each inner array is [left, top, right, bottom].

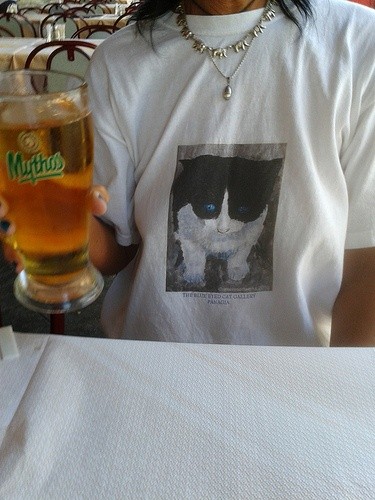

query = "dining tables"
[[1, 330, 375, 498]]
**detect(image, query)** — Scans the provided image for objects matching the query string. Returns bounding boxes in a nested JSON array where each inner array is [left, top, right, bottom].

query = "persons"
[[1, 0, 375, 347]]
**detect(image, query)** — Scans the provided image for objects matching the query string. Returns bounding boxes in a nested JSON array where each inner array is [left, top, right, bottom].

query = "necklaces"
[[176, 2, 277, 59], [203, 35, 254, 101]]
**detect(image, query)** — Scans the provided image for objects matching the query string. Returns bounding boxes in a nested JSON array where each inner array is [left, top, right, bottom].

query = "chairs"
[[1, 0, 140, 169]]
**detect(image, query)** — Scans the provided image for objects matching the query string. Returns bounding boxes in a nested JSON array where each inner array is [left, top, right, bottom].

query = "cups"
[[1, 67, 104, 315], [115, 3, 123, 17], [46, 23, 66, 43]]
